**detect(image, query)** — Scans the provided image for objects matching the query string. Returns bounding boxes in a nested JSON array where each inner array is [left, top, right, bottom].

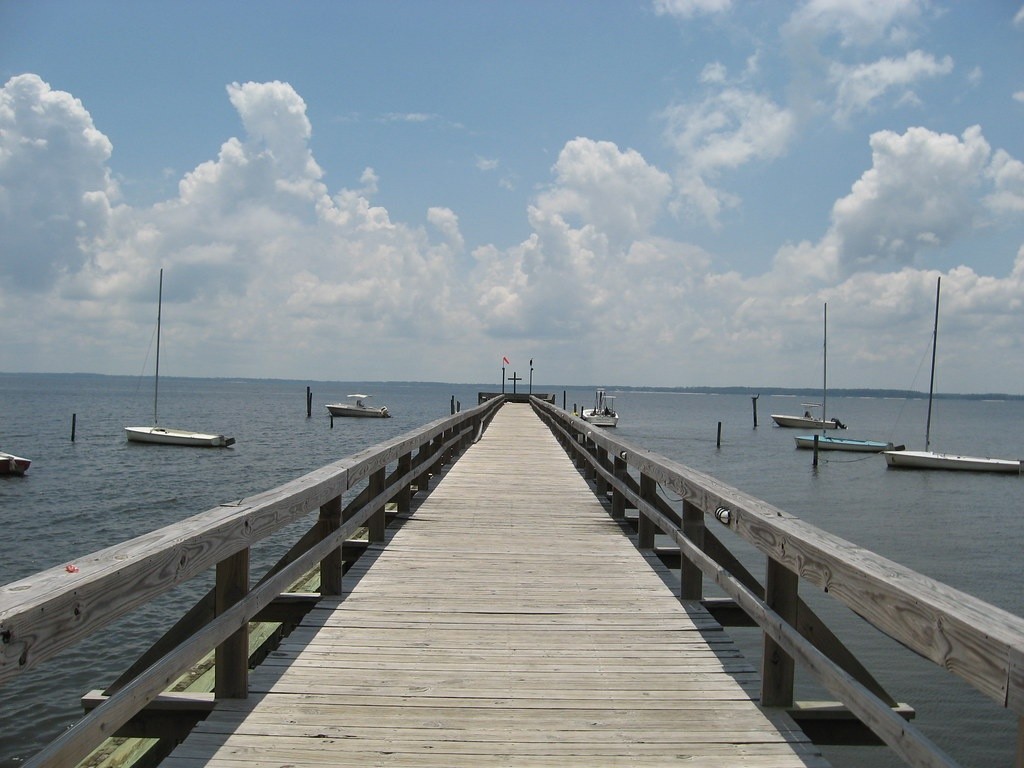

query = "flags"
[[504, 356, 510, 365]]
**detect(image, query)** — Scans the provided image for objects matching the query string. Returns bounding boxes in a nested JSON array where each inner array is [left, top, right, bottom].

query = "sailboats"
[[885, 282, 1022, 474], [122, 268, 237, 447], [793, 301, 907, 455]]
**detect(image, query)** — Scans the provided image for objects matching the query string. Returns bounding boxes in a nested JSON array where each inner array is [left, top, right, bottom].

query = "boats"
[[324, 394, 394, 418], [582, 387, 620, 428], [771, 404, 848, 430], [0, 451, 32, 475]]
